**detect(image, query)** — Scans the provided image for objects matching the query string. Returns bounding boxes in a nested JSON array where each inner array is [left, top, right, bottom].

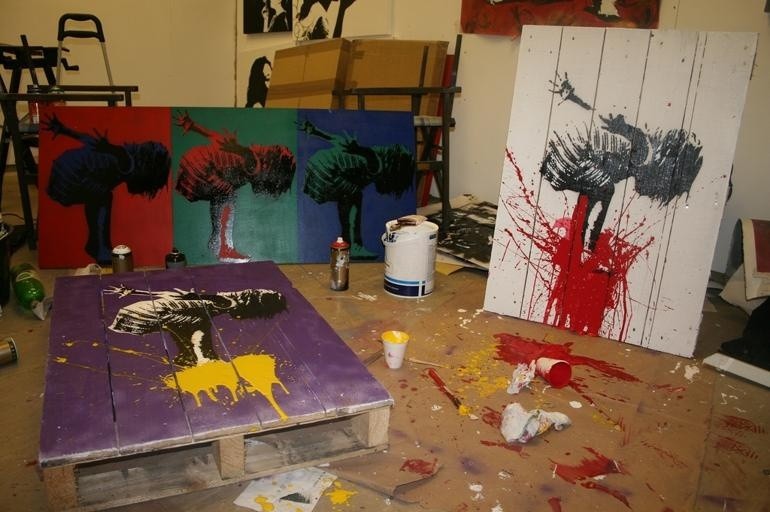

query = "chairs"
[[0, 44, 139, 252]]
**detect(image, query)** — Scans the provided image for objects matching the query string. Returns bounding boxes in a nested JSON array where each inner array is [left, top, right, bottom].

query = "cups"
[[534, 358, 574, 388], [381, 330, 410, 369], [0, 336, 19, 365]]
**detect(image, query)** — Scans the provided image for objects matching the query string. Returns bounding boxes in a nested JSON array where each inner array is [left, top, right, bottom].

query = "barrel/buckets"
[[382, 220, 438, 299]]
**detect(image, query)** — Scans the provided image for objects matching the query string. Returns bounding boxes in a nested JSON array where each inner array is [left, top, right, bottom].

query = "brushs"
[[390, 215, 428, 231]]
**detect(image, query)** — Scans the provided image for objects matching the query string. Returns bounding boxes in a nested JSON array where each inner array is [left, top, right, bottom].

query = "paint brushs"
[[404, 356, 444, 368], [428, 368, 472, 416]]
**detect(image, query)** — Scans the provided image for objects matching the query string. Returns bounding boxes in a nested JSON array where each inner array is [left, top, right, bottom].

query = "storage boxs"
[[264, 36, 450, 158]]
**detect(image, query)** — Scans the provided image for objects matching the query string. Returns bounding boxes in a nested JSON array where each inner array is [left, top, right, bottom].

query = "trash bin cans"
[[0, 221, 14, 307]]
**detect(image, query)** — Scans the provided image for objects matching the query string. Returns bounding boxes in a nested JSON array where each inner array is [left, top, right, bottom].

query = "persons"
[[540, 71, 704, 276], [168, 108, 297, 263], [259, 0, 290, 32], [40, 112, 171, 265], [102, 283, 287, 367], [244, 57, 273, 108], [292, 114, 415, 262], [292, 0, 341, 41]]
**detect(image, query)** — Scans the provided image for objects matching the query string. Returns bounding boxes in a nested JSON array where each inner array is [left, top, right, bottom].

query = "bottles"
[[112, 244, 133, 274], [10, 262, 44, 309], [27, 84, 65, 126], [329, 238, 349, 291], [165, 246, 185, 269]]
